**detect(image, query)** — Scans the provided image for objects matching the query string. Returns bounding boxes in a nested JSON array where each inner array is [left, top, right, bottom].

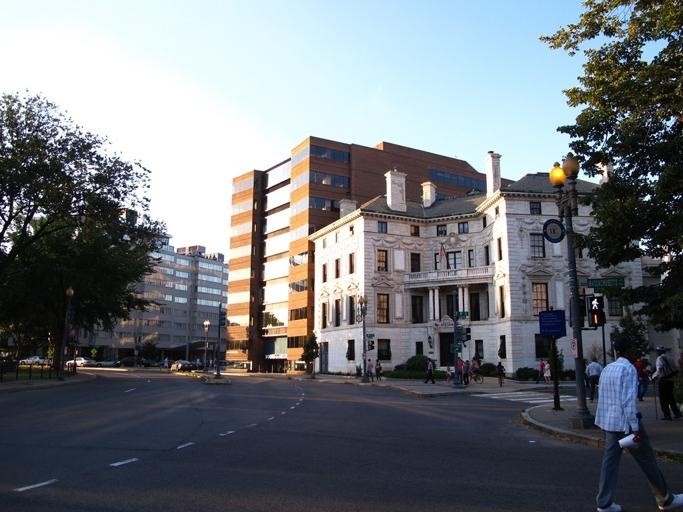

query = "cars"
[[18, 355, 46, 365], [171, 359, 194, 372], [65, 356, 161, 367]]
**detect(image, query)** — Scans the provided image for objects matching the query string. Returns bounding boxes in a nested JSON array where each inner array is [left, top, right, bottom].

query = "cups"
[[618, 434, 640, 448]]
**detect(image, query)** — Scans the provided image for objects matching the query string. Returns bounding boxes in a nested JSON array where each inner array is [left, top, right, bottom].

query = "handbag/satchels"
[[659, 355, 679, 378]]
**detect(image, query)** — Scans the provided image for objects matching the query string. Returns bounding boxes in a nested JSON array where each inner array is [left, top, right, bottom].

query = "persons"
[[213, 357, 217, 372], [584, 356, 603, 403], [374, 358, 382, 382], [647, 344, 682, 421], [592, 339, 682, 512], [635, 352, 651, 401], [446, 363, 451, 384], [365, 358, 374, 382], [423, 357, 435, 384], [542, 360, 550, 385], [163, 356, 168, 368], [457, 356, 481, 386], [535, 358, 544, 384], [495, 361, 506, 388]]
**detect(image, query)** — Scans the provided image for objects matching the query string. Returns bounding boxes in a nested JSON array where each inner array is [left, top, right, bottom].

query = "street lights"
[[203, 319, 211, 371], [359, 295, 369, 383], [549, 152, 595, 431]]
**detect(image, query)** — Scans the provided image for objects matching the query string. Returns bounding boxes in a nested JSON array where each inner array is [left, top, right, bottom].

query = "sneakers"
[[596, 501, 622, 512], [657, 493, 683, 510], [660, 412, 682, 421], [423, 378, 477, 385]]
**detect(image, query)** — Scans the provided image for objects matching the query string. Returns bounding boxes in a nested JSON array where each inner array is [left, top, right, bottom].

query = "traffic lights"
[[589, 296, 604, 327]]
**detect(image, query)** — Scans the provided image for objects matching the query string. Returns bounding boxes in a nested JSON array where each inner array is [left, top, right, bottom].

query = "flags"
[[439, 242, 447, 270]]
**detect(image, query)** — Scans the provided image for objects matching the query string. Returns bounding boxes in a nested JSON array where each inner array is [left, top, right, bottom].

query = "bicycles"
[[469, 370, 483, 384], [499, 369, 505, 387]]
